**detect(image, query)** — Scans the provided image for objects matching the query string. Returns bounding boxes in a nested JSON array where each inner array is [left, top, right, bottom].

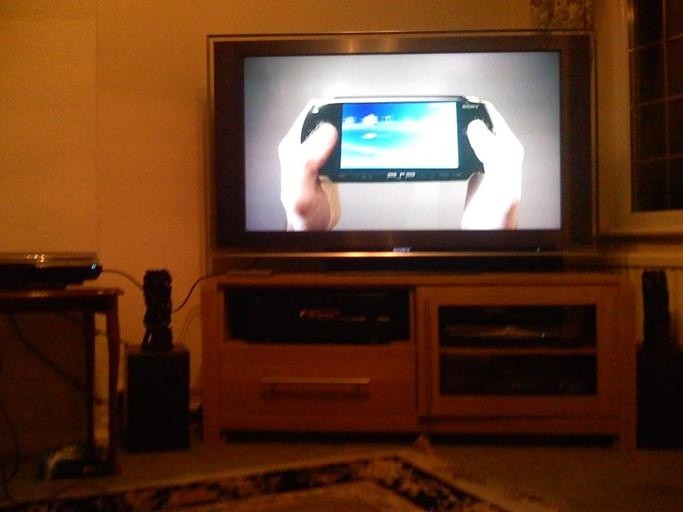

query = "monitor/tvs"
[[205, 31, 577, 272]]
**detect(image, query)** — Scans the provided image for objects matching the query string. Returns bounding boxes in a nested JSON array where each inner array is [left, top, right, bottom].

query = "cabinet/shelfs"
[[0, 285, 124, 457], [198, 270, 639, 466]]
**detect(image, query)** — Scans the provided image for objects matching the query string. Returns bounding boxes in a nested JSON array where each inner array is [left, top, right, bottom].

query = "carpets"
[[0, 446, 549, 512]]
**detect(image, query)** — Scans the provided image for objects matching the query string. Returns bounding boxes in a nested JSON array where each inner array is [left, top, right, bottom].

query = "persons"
[[274, 94, 527, 234]]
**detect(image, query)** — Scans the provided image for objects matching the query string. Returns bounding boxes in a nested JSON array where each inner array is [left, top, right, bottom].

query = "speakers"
[[124, 345, 190, 454], [637, 349, 683, 447]]
